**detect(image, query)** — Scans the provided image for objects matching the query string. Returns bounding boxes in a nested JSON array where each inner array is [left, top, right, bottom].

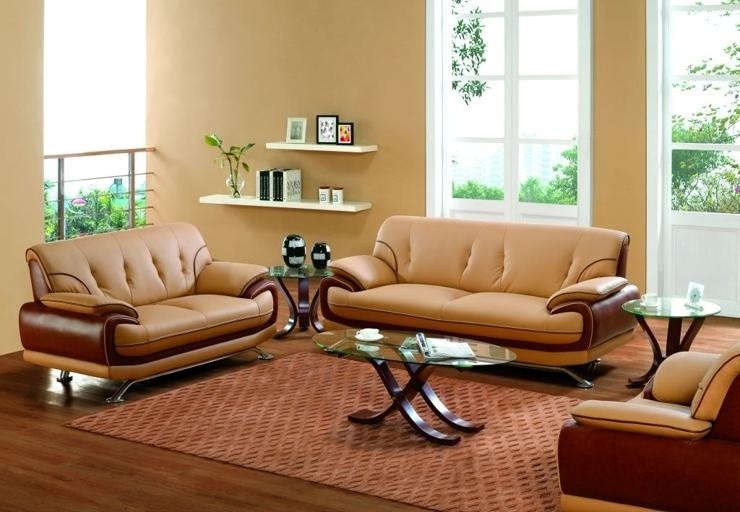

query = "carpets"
[[62, 351, 583, 512]]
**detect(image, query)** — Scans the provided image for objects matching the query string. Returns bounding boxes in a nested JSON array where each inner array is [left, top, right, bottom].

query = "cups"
[[356, 328, 379, 339], [331, 187, 345, 205], [357, 344, 378, 352], [318, 185, 331, 203], [640, 293, 658, 303]]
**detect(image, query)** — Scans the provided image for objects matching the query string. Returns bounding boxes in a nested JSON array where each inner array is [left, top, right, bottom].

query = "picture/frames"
[[337, 122, 354, 145], [285, 116, 307, 143], [316, 114, 339, 144]]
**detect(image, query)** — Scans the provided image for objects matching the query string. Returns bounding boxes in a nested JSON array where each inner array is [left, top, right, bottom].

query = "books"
[[256, 167, 302, 201], [399, 333, 476, 359]]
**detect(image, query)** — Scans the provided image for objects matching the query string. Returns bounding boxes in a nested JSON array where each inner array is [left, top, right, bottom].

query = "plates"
[[640, 301, 662, 308], [355, 333, 383, 342]]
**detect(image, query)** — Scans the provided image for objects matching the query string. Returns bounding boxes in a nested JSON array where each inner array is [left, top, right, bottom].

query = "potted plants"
[[204, 131, 255, 197]]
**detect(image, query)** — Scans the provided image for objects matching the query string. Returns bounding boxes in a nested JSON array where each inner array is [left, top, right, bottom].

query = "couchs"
[[19, 218, 278, 403], [557, 330, 740, 511], [319, 213, 645, 389]]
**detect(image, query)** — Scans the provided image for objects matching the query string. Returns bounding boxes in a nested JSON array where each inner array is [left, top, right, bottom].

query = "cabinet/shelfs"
[[200, 141, 379, 212]]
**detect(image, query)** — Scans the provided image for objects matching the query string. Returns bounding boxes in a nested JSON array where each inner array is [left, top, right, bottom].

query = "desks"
[[623, 296, 723, 386], [266, 265, 334, 339]]
